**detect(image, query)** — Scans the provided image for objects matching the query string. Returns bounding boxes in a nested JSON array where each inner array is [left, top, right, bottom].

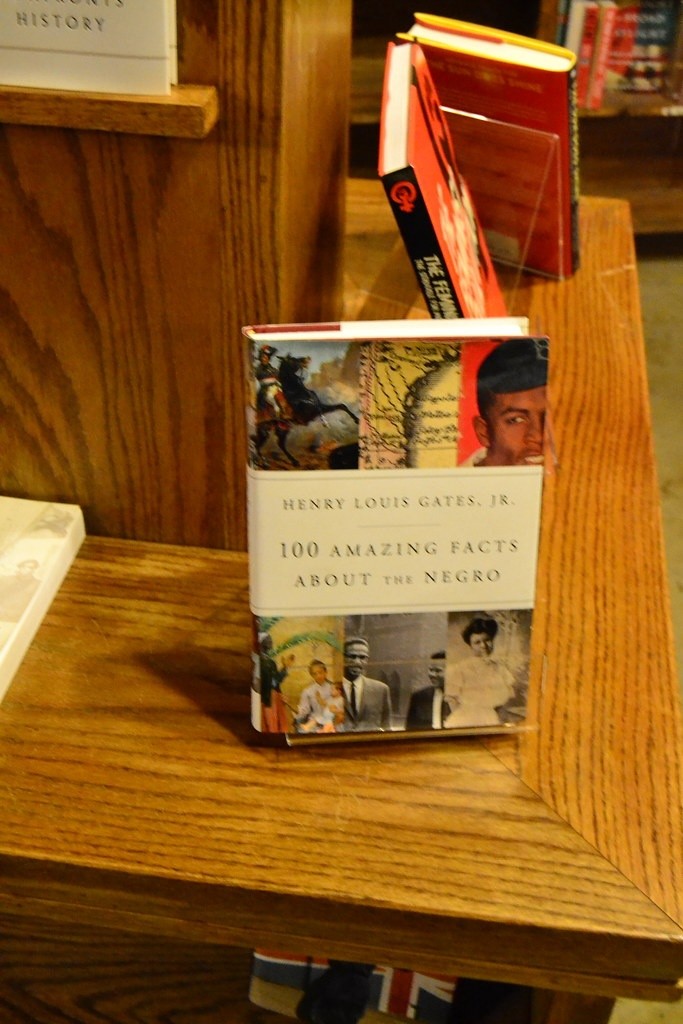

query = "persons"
[[407, 653, 445, 729], [343, 637, 392, 732], [460, 338, 549, 464], [445, 620, 515, 728], [255, 347, 300, 468]]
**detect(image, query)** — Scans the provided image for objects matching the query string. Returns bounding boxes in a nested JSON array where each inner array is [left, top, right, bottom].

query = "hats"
[[463, 617, 498, 646]]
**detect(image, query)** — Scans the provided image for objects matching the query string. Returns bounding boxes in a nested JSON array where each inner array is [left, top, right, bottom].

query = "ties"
[[351, 682, 358, 717]]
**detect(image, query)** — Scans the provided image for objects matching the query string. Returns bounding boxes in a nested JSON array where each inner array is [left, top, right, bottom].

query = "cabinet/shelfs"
[[0, 0, 683, 1024]]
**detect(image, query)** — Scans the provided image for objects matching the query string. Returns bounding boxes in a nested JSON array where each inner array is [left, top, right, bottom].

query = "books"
[[378, 44, 511, 320], [1, 0, 178, 98], [556, 0, 670, 111], [394, 14, 582, 282], [243, 317, 549, 733], [0, 494, 86, 705]]
